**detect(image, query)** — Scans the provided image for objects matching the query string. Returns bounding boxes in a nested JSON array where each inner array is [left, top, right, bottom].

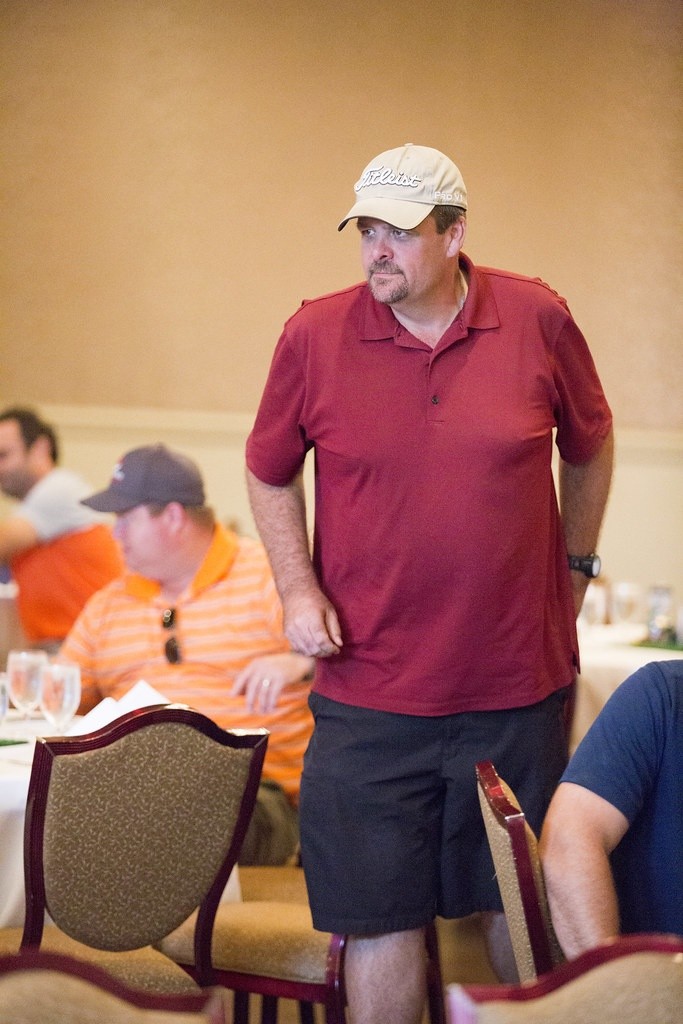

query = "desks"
[[0, 708, 243, 930], [568, 624, 683, 760]]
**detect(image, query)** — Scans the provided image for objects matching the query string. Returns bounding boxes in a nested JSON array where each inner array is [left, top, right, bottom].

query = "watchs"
[[567, 552, 601, 578]]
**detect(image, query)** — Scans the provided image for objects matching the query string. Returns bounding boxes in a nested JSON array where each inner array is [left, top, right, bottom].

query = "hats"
[[80, 442, 204, 511], [338, 142, 469, 231]]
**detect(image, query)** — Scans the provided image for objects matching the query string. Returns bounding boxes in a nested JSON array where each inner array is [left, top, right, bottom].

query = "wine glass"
[[6, 649, 49, 722], [578, 582, 643, 666], [36, 659, 81, 736]]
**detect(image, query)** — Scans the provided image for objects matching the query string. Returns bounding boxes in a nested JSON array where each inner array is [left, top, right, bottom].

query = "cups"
[[0, 672, 10, 729]]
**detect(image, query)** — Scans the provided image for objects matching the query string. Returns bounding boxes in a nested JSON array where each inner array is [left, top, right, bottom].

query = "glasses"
[[162, 609, 182, 665]]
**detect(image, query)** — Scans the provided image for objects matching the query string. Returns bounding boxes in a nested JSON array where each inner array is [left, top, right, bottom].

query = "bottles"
[[649, 581, 674, 642]]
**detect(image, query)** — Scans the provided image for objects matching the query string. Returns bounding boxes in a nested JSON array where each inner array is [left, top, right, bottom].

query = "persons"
[[537, 660, 682, 961], [9, 439, 314, 869], [0, 408, 122, 659], [244, 144, 613, 1024]]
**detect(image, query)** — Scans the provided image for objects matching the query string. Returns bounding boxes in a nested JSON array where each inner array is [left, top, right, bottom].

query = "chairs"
[[0, 702, 272, 997], [446, 933, 683, 1024], [472, 757, 564, 984], [0, 945, 224, 1024], [157, 898, 348, 1024]]
